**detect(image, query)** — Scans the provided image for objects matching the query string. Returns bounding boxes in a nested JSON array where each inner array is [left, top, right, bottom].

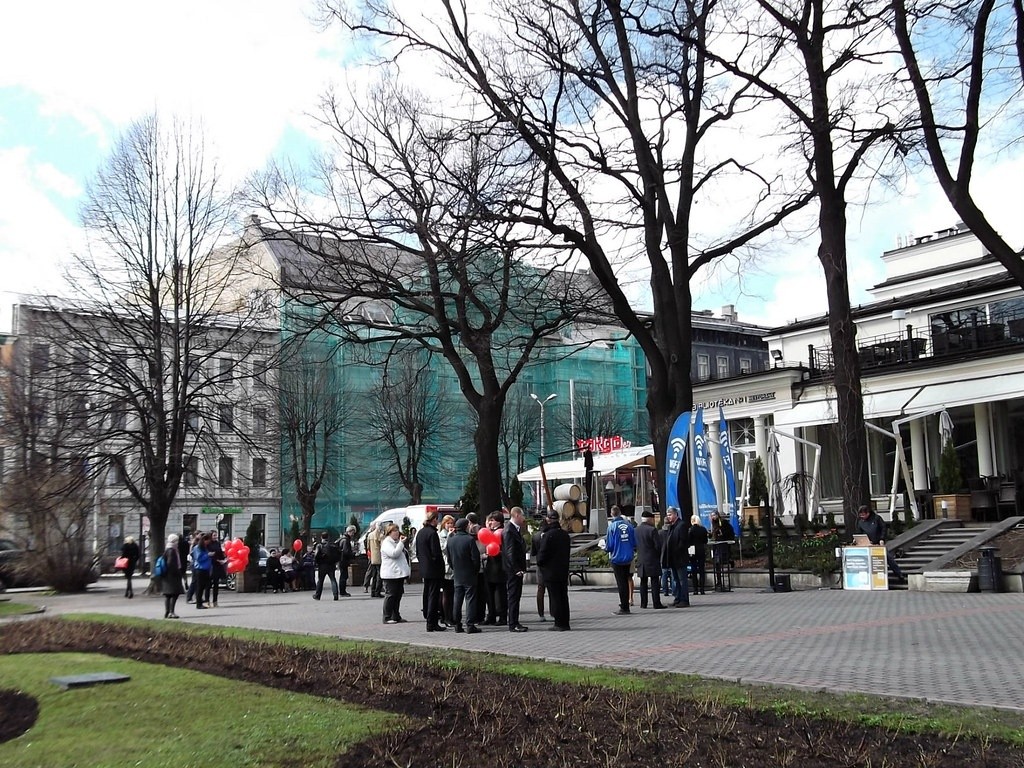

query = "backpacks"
[[154, 550, 168, 577], [318, 543, 334, 562]]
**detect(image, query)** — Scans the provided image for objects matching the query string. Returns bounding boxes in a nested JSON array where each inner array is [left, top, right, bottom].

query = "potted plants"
[[933, 436, 972, 522], [236, 519, 261, 592], [346, 513, 364, 586], [744, 455, 774, 526], [401, 516, 421, 584]]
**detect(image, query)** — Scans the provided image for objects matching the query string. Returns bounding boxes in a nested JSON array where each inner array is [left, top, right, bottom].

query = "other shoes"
[[423, 617, 508, 633], [187, 600, 218, 609], [165, 613, 178, 618], [540, 616, 546, 622], [333, 594, 339, 601], [548, 626, 564, 631], [386, 619, 397, 624], [611, 591, 706, 616], [340, 592, 351, 597], [313, 594, 319, 600], [899, 575, 904, 579], [273, 587, 305, 593], [400, 618, 407, 622], [364, 587, 385, 598]]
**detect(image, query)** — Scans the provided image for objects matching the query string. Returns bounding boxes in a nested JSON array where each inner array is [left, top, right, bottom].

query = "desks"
[[659, 540, 736, 594]]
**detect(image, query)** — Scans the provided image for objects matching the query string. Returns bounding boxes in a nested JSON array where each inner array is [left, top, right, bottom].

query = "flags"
[[666, 406, 741, 536]]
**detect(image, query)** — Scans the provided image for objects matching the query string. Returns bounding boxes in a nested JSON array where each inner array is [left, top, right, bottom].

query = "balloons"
[[486, 542, 500, 556], [294, 540, 302, 551], [492, 529, 504, 544], [477, 528, 492, 545], [224, 539, 250, 573]]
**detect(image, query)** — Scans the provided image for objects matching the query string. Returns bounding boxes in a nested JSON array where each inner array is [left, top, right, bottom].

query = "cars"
[[218, 543, 272, 591], [100, 549, 121, 574]]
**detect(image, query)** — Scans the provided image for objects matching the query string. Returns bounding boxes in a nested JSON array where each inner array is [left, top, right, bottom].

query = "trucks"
[[357, 503, 463, 563]]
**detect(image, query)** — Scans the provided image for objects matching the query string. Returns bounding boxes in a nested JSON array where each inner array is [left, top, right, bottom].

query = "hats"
[[547, 511, 559, 519], [642, 511, 655, 517], [454, 518, 469, 530], [168, 533, 179, 543]]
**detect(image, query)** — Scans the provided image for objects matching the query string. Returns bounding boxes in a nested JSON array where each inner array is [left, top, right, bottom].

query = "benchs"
[[525, 557, 591, 585]]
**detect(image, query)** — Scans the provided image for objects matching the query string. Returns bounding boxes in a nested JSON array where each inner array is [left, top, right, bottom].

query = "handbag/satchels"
[[114, 556, 129, 569], [292, 560, 300, 570], [688, 545, 695, 554]]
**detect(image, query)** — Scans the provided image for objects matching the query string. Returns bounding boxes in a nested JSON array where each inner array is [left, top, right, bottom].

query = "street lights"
[[138, 512, 148, 572], [530, 393, 557, 510]]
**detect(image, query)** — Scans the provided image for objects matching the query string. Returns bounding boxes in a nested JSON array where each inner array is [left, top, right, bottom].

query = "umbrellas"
[[767, 428, 821, 522], [892, 406, 954, 520]]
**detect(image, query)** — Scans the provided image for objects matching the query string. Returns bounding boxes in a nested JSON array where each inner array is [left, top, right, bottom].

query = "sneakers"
[[510, 624, 528, 632]]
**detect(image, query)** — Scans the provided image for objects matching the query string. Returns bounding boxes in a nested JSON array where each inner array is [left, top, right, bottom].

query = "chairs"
[[858, 318, 1024, 367], [903, 476, 1004, 521]]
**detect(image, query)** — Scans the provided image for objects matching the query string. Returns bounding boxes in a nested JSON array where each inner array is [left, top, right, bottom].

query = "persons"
[[531, 510, 570, 631], [364, 512, 508, 634], [265, 544, 314, 592], [160, 528, 225, 619], [711, 510, 734, 587], [311, 525, 356, 600], [856, 506, 906, 584], [687, 515, 708, 595], [501, 507, 529, 632], [600, 505, 689, 615], [121, 536, 140, 598]]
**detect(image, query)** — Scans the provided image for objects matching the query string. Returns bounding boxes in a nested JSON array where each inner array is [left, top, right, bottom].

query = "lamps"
[[771, 350, 783, 361], [892, 310, 906, 358]]
